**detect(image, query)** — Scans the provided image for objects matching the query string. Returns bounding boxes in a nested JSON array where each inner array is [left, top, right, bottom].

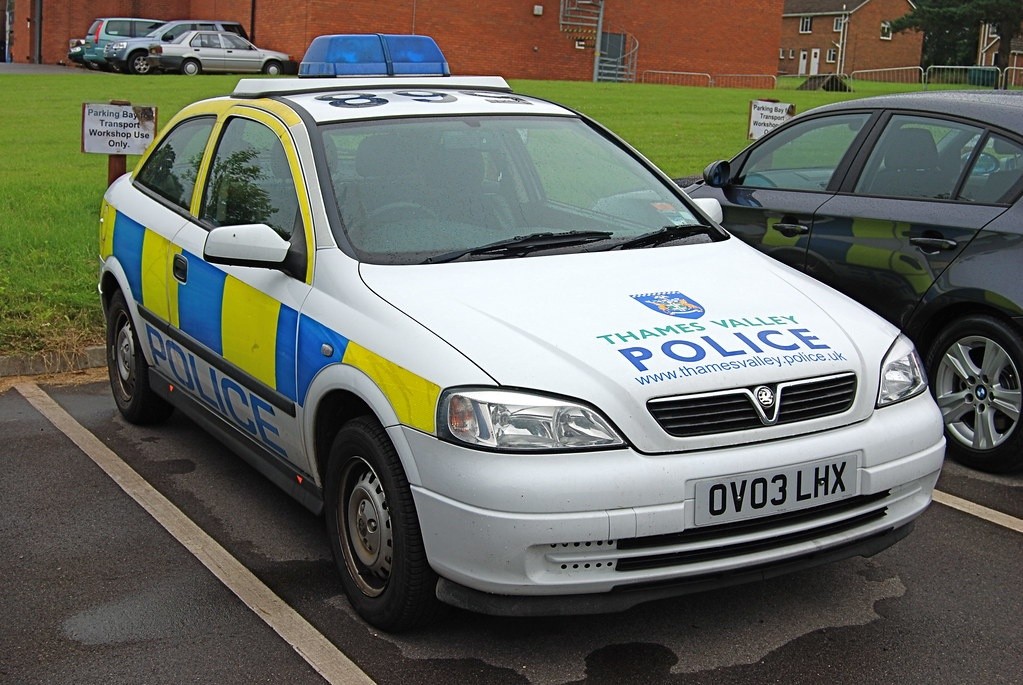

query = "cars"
[[68, 38, 100, 71], [146, 30, 296, 77], [92, 33, 947, 637], [588, 91, 1022, 477]]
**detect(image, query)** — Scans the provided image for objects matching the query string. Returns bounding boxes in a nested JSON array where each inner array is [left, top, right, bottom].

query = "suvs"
[[83, 16, 173, 72], [103, 19, 250, 75]]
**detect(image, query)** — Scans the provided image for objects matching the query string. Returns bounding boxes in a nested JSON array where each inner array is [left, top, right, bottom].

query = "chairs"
[[272, 131, 336, 211], [873, 129, 948, 196], [356, 134, 421, 223], [428, 144, 515, 231]]
